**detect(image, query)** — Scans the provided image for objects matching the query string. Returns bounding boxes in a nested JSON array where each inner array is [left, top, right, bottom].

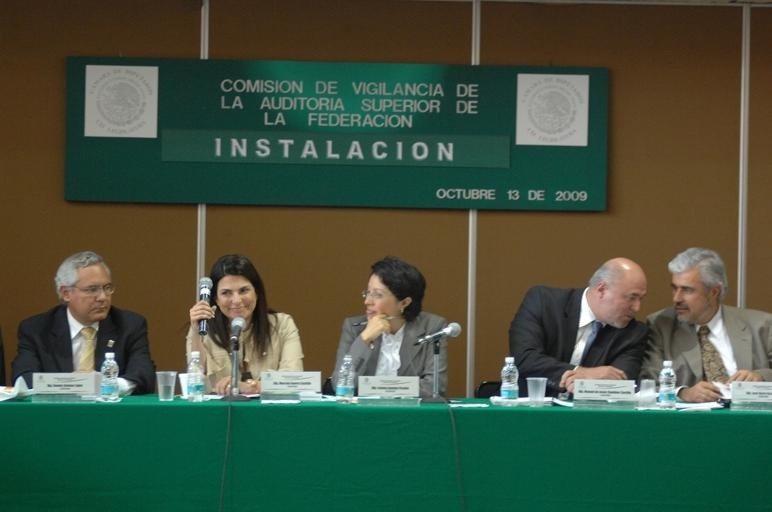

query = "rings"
[[227, 383, 231, 388]]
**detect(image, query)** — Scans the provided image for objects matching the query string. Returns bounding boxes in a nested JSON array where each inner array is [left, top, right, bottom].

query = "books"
[[488, 395, 554, 407]]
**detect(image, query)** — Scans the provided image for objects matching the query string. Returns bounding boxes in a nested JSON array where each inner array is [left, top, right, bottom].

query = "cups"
[[639, 378, 657, 411], [155, 370, 177, 402], [178, 373, 187, 399], [526, 376, 549, 408]]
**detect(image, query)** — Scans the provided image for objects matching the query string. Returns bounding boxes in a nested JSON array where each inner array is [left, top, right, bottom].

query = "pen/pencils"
[[352, 315, 395, 328]]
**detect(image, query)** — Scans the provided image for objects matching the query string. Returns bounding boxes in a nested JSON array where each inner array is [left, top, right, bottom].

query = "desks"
[[0, 387, 771, 512]]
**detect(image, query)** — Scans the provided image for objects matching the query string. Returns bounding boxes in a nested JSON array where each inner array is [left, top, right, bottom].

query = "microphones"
[[230, 317, 246, 342], [198, 277, 213, 336], [414, 322, 461, 345]]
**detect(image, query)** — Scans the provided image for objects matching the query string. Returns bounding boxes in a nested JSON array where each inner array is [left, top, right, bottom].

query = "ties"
[[76, 326, 97, 372], [579, 320, 602, 366], [698, 325, 729, 383]]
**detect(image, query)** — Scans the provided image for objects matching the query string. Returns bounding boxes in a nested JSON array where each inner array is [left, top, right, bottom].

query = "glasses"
[[70, 283, 117, 299], [360, 289, 384, 300]]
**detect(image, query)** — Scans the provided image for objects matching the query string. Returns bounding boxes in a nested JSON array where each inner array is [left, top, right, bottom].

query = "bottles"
[[336, 353, 357, 405], [100, 351, 120, 402], [657, 360, 678, 411], [187, 351, 205, 403], [498, 355, 521, 409]]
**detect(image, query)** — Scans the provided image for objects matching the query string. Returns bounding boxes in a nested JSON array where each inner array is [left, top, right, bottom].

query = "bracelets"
[[191, 323, 199, 330], [247, 377, 260, 393]]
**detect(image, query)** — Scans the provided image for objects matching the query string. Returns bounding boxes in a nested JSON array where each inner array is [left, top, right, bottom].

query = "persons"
[[325, 255, 451, 402], [9, 249, 158, 396], [631, 245, 772, 402], [490, 257, 651, 399], [180, 255, 306, 397]]
